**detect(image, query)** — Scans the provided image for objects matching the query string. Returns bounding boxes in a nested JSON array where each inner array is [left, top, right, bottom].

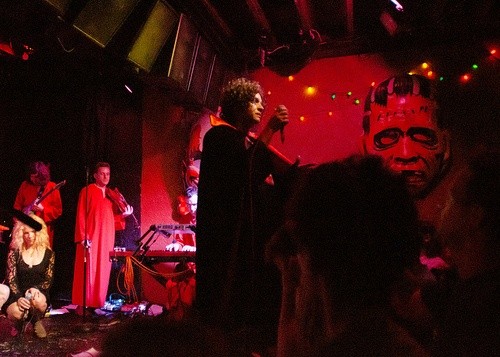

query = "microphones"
[[277, 105, 287, 143], [23, 293, 31, 319]]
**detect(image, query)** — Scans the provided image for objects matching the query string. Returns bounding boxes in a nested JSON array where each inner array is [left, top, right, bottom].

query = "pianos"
[[108, 249, 196, 301]]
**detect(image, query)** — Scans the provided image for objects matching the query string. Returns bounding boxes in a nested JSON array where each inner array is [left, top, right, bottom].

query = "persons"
[[195, 77, 500, 357], [0, 160, 134, 339]]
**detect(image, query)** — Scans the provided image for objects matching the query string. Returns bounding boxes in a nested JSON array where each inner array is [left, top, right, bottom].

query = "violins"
[[109, 187, 140, 229]]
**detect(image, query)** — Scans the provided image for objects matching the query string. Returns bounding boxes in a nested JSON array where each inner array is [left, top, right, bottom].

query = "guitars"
[[22, 180, 67, 215]]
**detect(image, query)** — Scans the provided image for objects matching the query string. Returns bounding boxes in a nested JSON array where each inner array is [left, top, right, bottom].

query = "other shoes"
[[72, 305, 91, 316], [94, 308, 107, 315], [32, 320, 48, 340]]
